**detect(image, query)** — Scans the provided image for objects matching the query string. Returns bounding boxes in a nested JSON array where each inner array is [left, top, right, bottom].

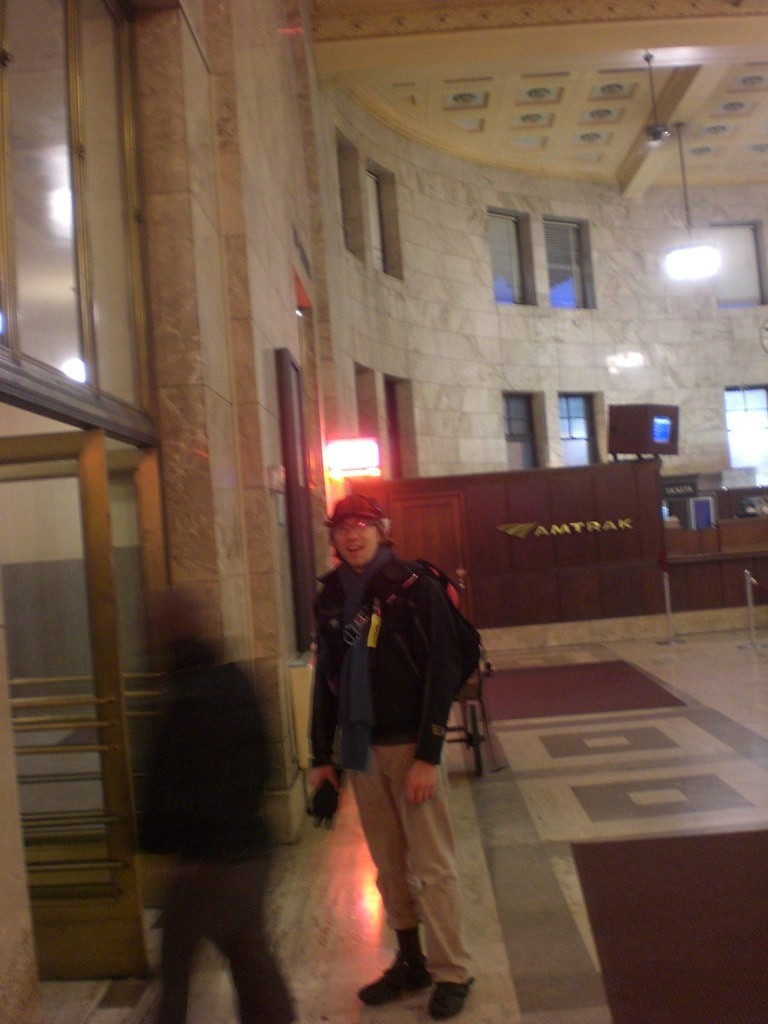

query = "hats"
[[323, 493, 384, 526]]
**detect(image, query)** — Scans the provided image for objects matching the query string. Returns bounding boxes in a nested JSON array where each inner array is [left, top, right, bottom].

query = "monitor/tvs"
[[654, 416, 673, 443]]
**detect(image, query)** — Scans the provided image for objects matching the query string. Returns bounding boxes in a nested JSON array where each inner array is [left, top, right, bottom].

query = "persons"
[[304, 493, 481, 1020], [735, 498, 759, 517], [134, 586, 298, 1024]]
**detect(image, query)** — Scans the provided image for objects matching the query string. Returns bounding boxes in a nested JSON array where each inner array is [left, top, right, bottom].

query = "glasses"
[[331, 520, 375, 533]]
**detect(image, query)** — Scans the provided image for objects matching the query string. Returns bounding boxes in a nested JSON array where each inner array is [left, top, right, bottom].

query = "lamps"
[[642, 53, 669, 145], [662, 122, 721, 281]]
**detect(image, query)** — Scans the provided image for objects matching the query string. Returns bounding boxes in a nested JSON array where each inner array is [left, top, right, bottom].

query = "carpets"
[[464, 658, 685, 721], [571, 830, 768, 1024]]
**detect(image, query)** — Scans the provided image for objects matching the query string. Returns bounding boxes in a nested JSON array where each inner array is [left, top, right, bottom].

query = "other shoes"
[[428, 974, 475, 1019], [359, 949, 431, 1006]]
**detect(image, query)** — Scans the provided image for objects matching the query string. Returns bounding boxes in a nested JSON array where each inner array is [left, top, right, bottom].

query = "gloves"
[[304, 766, 344, 831]]
